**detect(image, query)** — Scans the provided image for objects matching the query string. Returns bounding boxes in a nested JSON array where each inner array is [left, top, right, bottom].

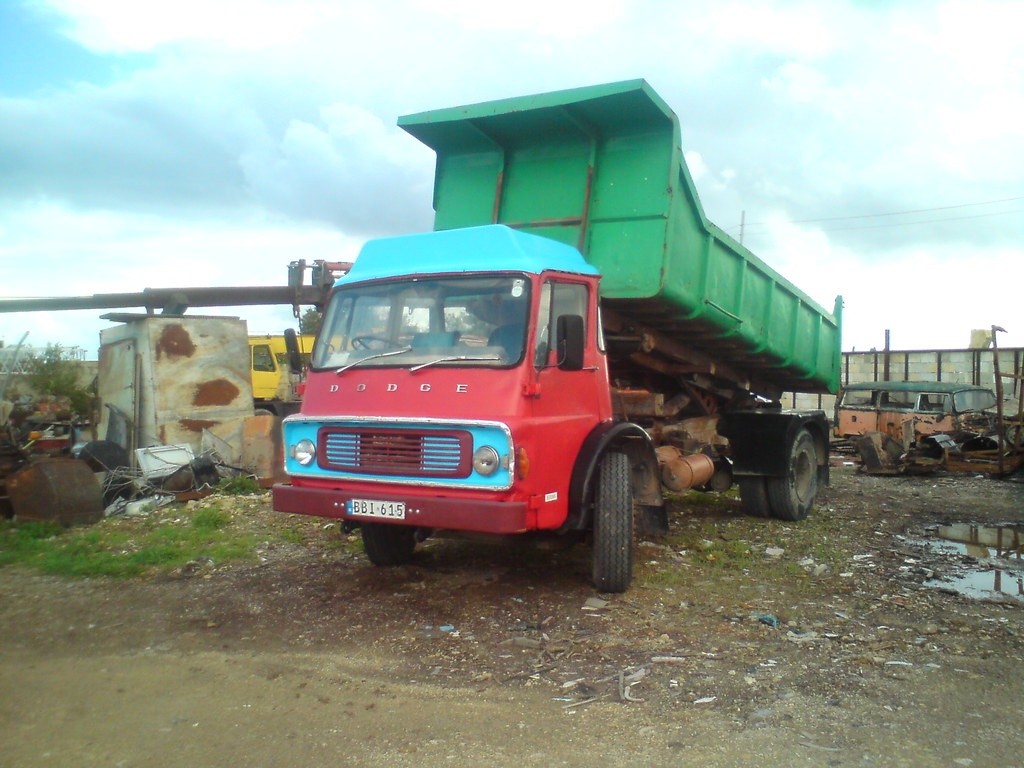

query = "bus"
[[832, 380, 998, 443]]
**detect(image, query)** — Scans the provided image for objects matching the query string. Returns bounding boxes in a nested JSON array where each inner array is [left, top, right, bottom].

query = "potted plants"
[[36, 394, 56, 417], [29, 427, 41, 438], [33, 353, 78, 419]]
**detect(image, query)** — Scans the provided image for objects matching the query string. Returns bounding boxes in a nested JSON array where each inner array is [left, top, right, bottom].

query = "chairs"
[[412, 330, 461, 350], [487, 322, 549, 365]]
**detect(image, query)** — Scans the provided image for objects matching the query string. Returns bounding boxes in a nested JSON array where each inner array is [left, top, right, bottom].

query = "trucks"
[[248, 332, 362, 418], [269, 76, 845, 595]]
